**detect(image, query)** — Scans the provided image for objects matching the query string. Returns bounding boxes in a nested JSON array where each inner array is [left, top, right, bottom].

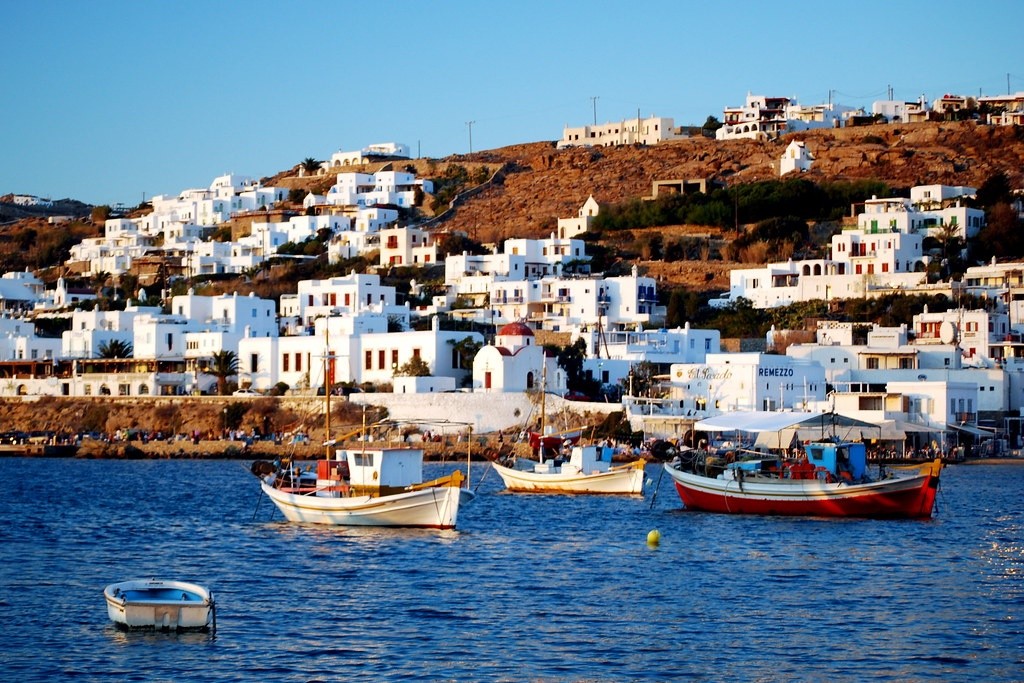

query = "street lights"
[[590, 96, 600, 126], [465, 121, 475, 153]]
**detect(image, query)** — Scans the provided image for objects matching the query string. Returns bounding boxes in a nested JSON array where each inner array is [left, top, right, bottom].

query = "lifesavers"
[[779, 462, 795, 480], [813, 466, 831, 484]]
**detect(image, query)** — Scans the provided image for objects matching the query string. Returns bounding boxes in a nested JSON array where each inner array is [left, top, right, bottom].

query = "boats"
[[250, 310, 476, 531], [663, 411, 942, 521], [104, 579, 215, 629], [491, 346, 648, 494]]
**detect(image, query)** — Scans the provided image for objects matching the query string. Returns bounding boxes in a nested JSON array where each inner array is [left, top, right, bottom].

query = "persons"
[[10, 426, 308, 448], [403, 429, 409, 442], [553, 433, 688, 463], [457, 430, 462, 443], [498, 429, 503, 442], [337, 385, 343, 396], [422, 429, 440, 442], [864, 441, 965, 459]]
